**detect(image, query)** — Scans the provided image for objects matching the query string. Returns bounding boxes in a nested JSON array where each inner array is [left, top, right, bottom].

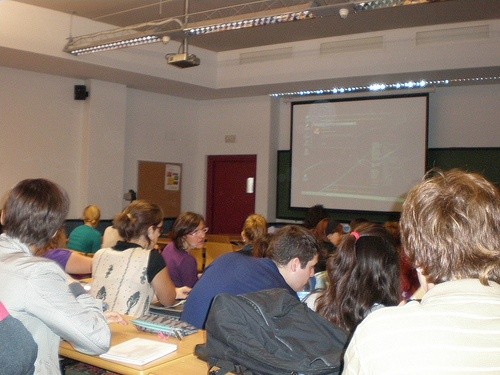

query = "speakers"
[[73, 85, 86, 100]]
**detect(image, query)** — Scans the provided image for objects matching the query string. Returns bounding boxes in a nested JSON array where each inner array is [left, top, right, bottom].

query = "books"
[[99, 337, 178, 365]]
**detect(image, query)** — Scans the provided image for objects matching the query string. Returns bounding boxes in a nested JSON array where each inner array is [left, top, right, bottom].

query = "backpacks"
[[194, 287, 351, 375]]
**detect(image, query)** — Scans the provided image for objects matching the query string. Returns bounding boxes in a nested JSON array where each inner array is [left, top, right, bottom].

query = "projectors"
[[167, 54, 200, 68]]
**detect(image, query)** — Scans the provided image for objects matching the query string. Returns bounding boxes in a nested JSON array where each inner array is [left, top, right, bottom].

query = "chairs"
[[205, 242, 234, 268]]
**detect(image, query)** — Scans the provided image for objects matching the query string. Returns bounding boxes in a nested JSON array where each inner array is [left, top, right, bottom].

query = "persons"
[[0, 177, 111, 375], [0, 200, 420, 375], [342, 169, 500, 375]]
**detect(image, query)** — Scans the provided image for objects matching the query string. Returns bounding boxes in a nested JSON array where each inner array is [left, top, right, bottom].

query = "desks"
[[158, 232, 242, 244], [59, 314, 236, 375]]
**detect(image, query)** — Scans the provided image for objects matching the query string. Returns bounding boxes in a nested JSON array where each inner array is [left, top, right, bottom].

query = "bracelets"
[[406, 299, 421, 302]]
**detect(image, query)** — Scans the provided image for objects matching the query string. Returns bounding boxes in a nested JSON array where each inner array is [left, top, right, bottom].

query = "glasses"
[[185, 227, 209, 237], [155, 226, 165, 232]]
